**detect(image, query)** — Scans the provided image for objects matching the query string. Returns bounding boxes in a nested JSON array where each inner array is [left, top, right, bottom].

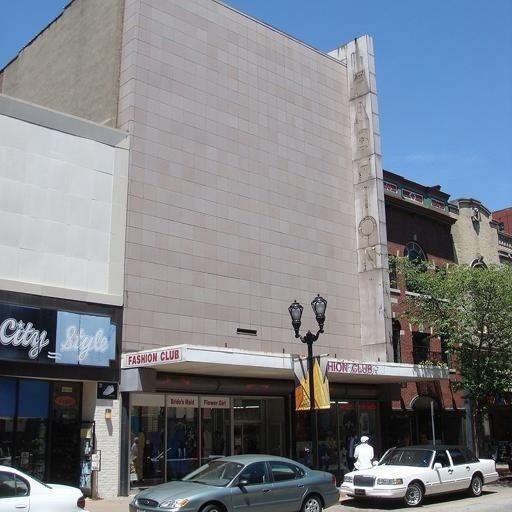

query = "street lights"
[[287, 292, 329, 471]]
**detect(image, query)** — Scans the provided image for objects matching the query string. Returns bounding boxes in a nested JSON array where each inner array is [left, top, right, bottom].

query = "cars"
[[340, 443, 502, 507], [0, 465, 89, 512], [129, 454, 342, 512]]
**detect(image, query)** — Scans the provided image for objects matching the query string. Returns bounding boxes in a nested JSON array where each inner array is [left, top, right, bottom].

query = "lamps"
[[105, 409, 112, 419]]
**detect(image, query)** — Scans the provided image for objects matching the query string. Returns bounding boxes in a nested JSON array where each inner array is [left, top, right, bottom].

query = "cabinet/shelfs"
[[80, 421, 95, 495]]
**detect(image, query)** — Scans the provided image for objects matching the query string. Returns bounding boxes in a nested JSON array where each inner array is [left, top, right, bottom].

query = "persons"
[[353, 436, 374, 470]]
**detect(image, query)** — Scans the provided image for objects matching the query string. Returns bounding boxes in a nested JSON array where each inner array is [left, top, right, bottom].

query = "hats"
[[360, 435, 369, 443]]
